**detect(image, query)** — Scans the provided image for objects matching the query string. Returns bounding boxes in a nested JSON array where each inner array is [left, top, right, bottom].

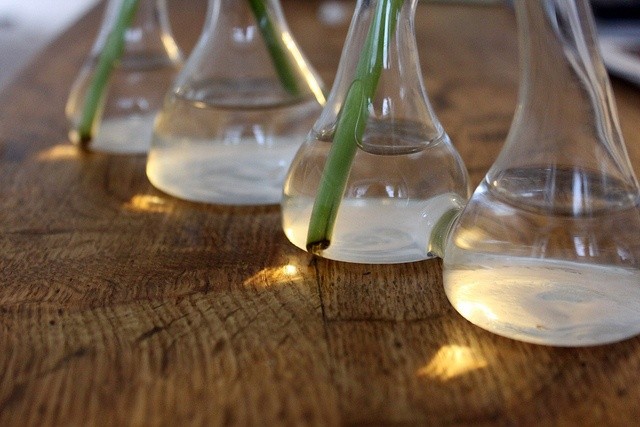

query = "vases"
[[145, 0, 327, 205], [281, 0, 471, 265], [442, 1, 640, 348], [63, 0, 184, 156]]
